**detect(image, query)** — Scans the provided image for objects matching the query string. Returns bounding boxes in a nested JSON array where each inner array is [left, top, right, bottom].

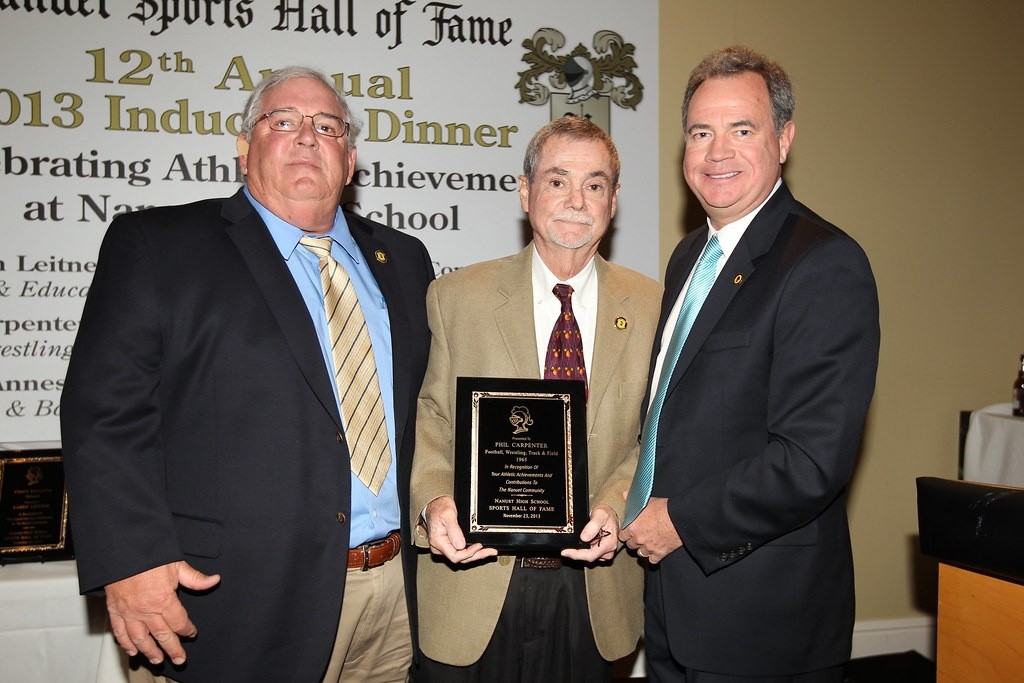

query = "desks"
[[955, 394, 1024, 486]]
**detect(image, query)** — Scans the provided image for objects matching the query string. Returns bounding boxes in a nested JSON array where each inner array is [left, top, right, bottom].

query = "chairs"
[[933, 559, 1024, 683], [917, 478, 1024, 606]]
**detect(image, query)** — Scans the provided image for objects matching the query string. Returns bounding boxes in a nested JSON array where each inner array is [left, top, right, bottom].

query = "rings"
[[637, 548, 649, 558]]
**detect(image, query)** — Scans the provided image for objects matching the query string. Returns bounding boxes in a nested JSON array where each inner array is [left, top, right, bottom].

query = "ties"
[[621, 233, 724, 528], [544, 284, 590, 404], [299, 236, 392, 495]]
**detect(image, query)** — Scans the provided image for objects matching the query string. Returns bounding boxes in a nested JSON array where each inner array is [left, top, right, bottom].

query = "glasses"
[[250, 110, 351, 138]]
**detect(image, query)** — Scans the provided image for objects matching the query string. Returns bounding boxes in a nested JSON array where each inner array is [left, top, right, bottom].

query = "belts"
[[515, 557, 579, 570], [347, 531, 401, 571]]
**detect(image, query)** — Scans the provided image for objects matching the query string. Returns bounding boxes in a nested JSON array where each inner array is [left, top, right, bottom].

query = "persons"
[[56, 65, 437, 683], [617, 46, 883, 683], [406, 115, 669, 683]]
